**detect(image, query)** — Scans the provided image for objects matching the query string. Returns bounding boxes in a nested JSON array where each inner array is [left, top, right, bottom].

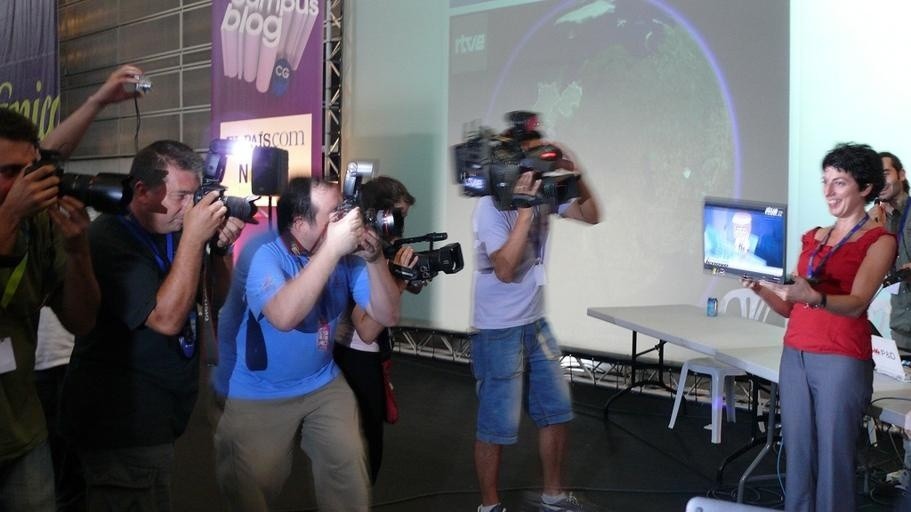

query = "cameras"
[[194, 138, 261, 224], [133, 73, 152, 95], [23, 149, 133, 216], [338, 159, 403, 253]]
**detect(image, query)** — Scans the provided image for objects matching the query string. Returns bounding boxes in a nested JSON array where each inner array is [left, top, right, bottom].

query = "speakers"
[[251, 147, 288, 196]]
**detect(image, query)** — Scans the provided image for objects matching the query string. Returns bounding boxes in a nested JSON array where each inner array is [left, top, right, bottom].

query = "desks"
[[583, 302, 786, 483], [716, 339, 909, 492]]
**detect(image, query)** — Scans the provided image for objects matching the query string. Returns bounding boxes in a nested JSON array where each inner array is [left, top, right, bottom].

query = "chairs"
[[856, 301, 894, 445], [662, 285, 772, 445]]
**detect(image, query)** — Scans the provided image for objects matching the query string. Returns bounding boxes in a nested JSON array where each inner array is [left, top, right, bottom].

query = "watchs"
[[810, 293, 828, 311]]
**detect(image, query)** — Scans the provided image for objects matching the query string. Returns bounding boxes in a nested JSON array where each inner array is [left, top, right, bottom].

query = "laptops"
[[702, 195, 817, 285]]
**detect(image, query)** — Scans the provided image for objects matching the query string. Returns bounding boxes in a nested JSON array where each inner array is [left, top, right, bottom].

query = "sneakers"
[[477, 502, 511, 512], [531, 491, 585, 512]]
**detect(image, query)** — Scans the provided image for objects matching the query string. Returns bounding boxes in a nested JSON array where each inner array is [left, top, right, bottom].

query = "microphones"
[[874, 195, 880, 223]]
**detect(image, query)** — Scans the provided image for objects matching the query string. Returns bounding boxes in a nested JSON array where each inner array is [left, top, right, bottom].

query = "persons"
[[467, 126, 601, 510], [35, 64, 145, 160], [868, 151, 911, 489], [336, 177, 418, 491], [741, 142, 896, 510], [61, 140, 247, 510], [1, 108, 101, 511], [211, 176, 374, 510]]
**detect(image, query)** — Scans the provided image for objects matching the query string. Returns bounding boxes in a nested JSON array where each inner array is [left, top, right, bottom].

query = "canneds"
[[707, 297, 718, 317]]
